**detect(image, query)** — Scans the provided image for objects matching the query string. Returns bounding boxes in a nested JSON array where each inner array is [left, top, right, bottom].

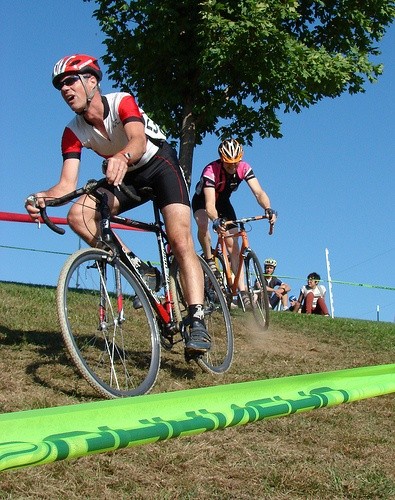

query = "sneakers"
[[186, 321, 211, 353], [133, 262, 163, 310], [204, 257, 222, 278], [237, 294, 251, 308]]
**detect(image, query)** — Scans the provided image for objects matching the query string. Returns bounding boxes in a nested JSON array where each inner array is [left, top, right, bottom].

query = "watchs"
[[120, 151, 131, 164]]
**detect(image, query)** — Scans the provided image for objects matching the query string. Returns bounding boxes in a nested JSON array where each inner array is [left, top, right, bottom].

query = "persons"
[[254, 259, 290, 311], [289, 272, 329, 316], [24, 54, 213, 351], [192, 138, 278, 308]]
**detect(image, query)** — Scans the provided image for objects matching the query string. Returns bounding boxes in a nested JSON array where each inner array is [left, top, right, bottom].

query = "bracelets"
[[282, 288, 285, 292]]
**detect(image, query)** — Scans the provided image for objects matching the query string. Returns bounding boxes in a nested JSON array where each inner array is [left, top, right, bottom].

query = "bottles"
[[127, 251, 149, 276]]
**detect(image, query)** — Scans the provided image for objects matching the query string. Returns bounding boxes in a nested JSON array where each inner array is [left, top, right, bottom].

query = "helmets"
[[218, 139, 243, 163], [289, 295, 296, 301], [51, 54, 102, 89], [264, 258, 277, 267]]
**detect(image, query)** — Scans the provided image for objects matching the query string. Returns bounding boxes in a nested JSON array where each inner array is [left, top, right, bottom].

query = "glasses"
[[55, 74, 91, 90]]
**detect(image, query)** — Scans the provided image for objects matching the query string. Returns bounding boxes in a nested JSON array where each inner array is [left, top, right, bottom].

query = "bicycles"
[[177, 210, 275, 332], [26, 160, 235, 401]]
